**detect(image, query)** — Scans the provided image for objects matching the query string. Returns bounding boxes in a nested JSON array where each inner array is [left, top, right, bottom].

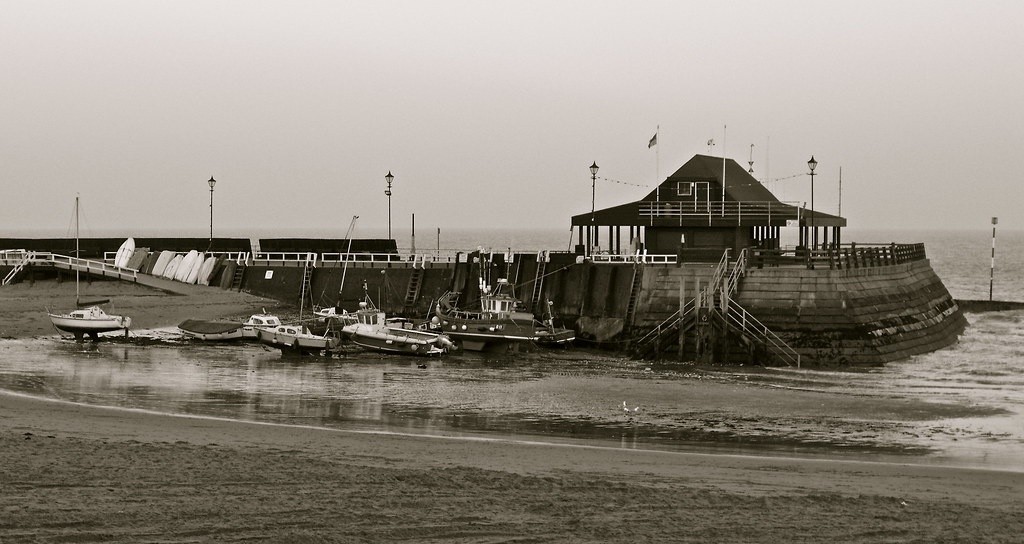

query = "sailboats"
[[252, 251, 342, 349], [311, 216, 358, 323], [47, 195, 132, 333]]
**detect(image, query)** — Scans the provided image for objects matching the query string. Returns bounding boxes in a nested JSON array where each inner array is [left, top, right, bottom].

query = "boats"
[[114, 236, 237, 291], [176, 313, 244, 340], [341, 313, 458, 357], [435, 246, 575, 350], [240, 314, 282, 338]]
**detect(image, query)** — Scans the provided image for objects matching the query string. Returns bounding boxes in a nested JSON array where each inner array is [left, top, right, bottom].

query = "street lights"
[[589, 160, 600, 252], [807, 155, 818, 250], [385, 171, 394, 239], [207, 176, 217, 250]]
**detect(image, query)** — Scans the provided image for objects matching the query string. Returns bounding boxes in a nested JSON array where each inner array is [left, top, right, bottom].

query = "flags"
[[648, 133, 657, 148]]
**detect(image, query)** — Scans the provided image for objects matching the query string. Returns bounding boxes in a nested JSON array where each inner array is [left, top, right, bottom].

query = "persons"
[[772, 244, 780, 267], [757, 242, 765, 269]]
